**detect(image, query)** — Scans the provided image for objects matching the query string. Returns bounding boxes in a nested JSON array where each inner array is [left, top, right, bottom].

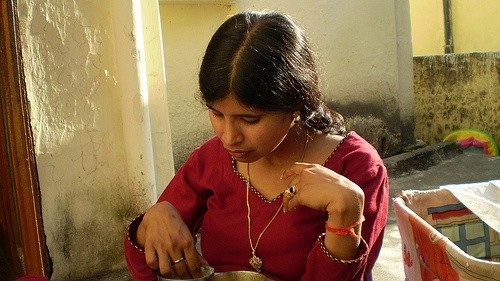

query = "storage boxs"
[[393, 180, 500, 281]]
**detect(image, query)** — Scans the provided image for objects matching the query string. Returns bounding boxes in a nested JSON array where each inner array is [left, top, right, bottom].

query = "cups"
[[157, 265, 214, 281]]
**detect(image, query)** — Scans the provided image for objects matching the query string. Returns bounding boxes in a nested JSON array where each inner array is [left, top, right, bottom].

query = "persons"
[[124, 9, 392, 281]]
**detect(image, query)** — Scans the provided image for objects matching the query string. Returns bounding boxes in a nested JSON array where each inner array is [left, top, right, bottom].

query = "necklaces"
[[242, 125, 311, 271]]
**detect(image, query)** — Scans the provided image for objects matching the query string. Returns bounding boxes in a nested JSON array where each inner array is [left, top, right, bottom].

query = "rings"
[[282, 185, 297, 199], [172, 257, 184, 264]]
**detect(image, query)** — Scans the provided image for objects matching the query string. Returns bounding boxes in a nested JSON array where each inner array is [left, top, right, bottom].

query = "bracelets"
[[323, 214, 367, 242]]
